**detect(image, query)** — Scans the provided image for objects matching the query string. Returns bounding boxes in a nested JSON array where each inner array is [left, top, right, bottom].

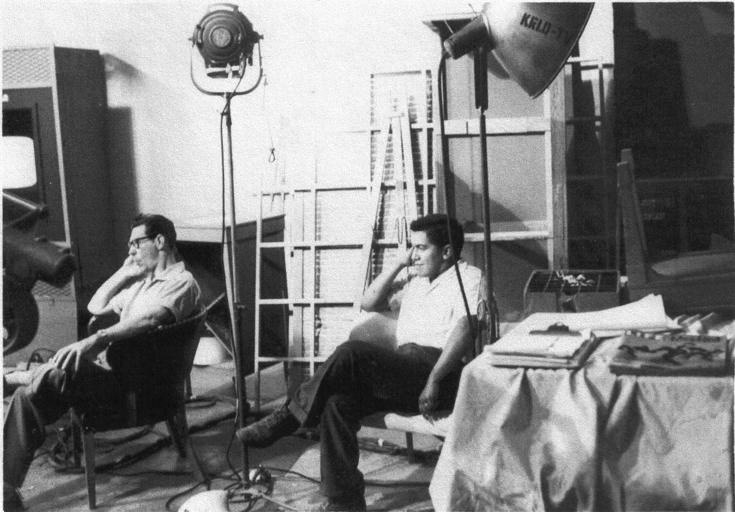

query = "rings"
[[424, 401, 428, 406]]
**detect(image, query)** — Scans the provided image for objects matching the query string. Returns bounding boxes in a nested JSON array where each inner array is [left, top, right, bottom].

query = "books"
[[610, 329, 730, 377], [489, 328, 601, 367]]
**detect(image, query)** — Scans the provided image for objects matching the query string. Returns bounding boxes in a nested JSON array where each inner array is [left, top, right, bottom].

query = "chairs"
[[67, 302, 213, 510]]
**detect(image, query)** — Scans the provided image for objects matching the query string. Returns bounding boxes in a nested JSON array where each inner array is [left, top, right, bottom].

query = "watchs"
[[95, 328, 113, 349]]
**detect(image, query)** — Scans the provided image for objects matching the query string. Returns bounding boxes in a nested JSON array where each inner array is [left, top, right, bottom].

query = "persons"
[[234, 214, 487, 512], [4, 215, 199, 511]]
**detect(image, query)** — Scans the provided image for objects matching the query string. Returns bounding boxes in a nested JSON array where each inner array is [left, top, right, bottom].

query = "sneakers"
[[235, 401, 301, 450]]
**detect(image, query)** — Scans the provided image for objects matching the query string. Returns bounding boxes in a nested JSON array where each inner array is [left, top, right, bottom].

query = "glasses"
[[126, 235, 150, 250]]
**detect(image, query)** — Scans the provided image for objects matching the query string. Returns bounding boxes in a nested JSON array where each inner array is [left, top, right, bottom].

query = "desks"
[[341, 291, 497, 464], [460, 310, 735, 512]]
